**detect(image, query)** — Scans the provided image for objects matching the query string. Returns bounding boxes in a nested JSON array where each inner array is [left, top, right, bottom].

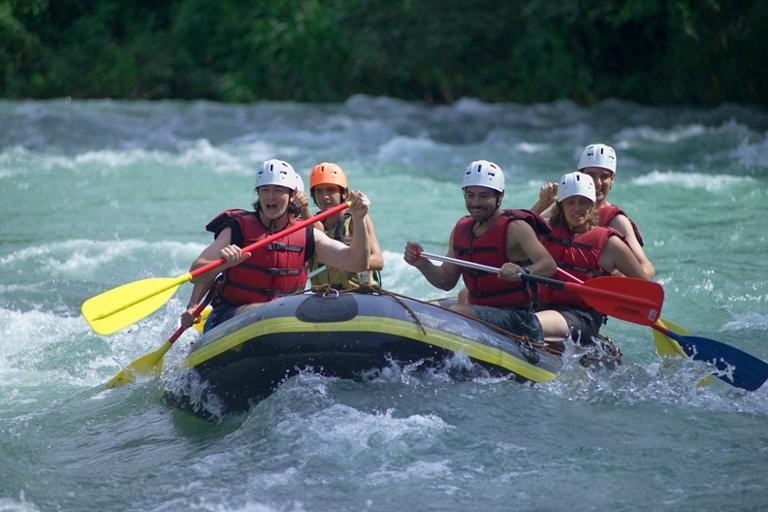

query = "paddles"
[[81, 203, 352, 335], [106, 275, 224, 388], [411, 250, 662, 326], [556, 267, 767, 390], [195, 261, 329, 335]]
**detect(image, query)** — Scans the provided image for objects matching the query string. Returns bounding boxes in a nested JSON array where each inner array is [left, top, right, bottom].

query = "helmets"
[[578, 144, 617, 175], [309, 162, 348, 192], [256, 159, 304, 193], [557, 171, 596, 204], [461, 160, 505, 193]]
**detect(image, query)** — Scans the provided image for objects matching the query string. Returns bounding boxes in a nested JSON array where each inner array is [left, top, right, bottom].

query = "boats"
[[156, 287, 623, 431]]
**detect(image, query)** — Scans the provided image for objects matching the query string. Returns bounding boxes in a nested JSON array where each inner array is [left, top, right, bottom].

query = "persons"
[[404, 160, 557, 345], [530, 143, 656, 280], [534, 171, 650, 346], [181, 159, 384, 334]]
